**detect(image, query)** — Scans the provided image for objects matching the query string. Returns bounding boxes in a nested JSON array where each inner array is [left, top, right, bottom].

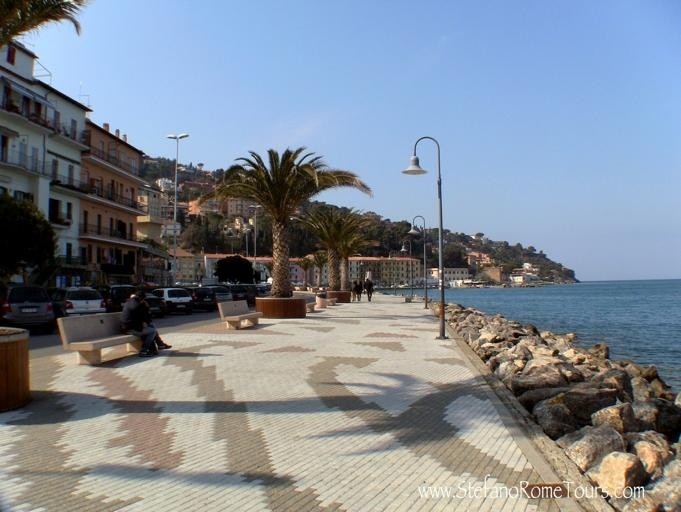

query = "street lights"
[[400, 240, 413, 296], [402, 135, 450, 341], [407, 216, 428, 310], [166, 132, 188, 282], [248, 203, 263, 256]]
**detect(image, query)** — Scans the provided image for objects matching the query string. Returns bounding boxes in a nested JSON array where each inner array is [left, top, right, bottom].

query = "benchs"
[[56, 312, 140, 366], [217, 300, 264, 329]]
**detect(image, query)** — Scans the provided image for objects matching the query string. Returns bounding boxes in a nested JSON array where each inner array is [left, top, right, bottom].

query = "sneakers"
[[138, 348, 158, 357], [158, 343, 172, 350]]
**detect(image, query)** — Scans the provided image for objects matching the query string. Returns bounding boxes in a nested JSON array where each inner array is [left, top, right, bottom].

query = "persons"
[[366, 278, 374, 302], [352, 280, 358, 301], [123, 289, 160, 359], [131, 293, 173, 355], [357, 281, 363, 301]]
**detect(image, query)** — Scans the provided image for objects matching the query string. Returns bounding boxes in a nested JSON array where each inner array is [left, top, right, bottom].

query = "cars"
[[2, 283, 271, 330]]
